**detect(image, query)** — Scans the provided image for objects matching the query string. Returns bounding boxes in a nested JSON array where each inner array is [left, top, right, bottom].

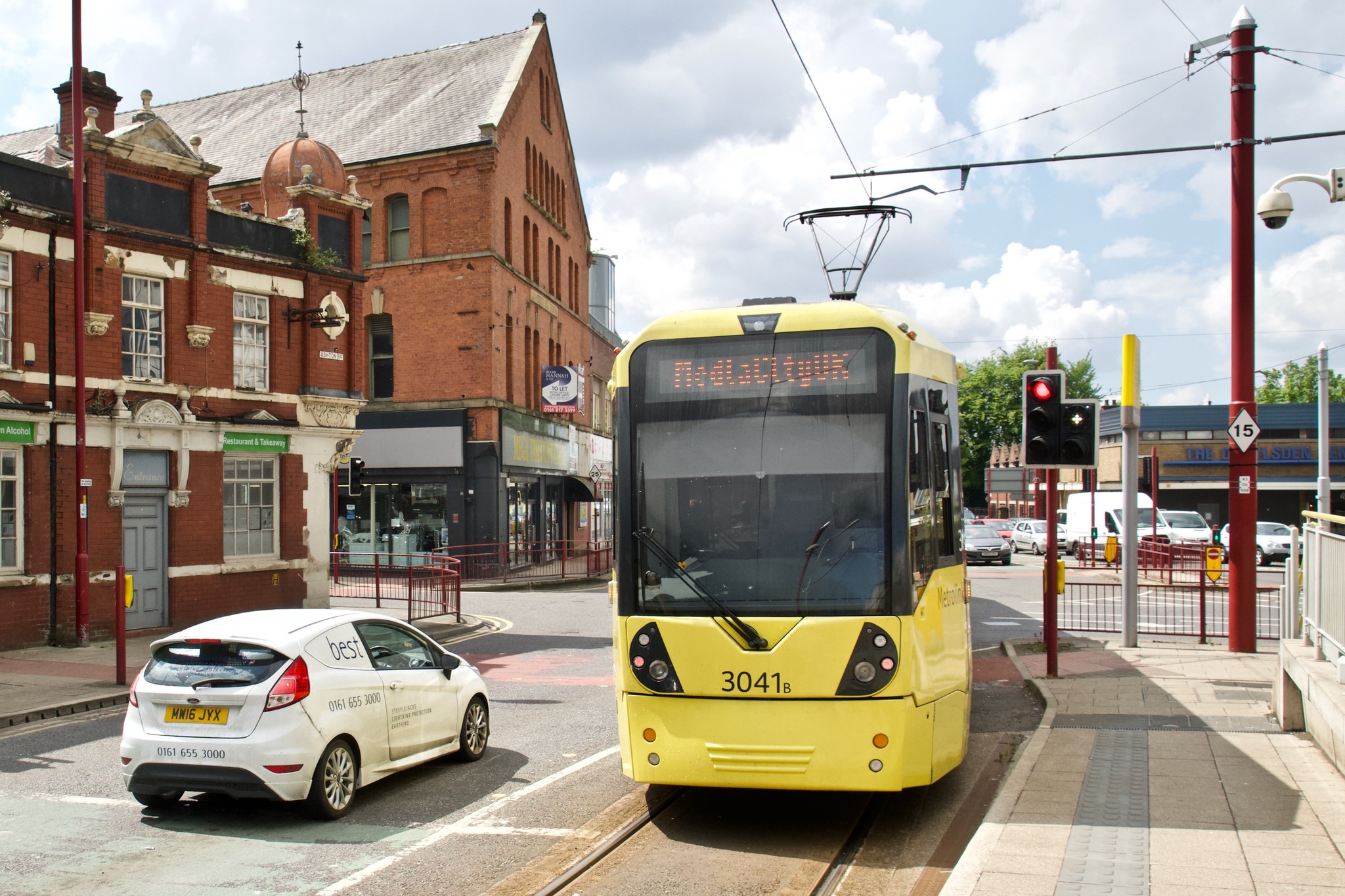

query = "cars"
[[1219, 521, 1304, 567], [961, 507, 1067, 565], [1161, 511, 1214, 560], [119, 609, 492, 821]]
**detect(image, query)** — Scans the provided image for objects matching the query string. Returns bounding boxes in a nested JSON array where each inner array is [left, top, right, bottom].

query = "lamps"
[[240, 202, 253, 212], [363, 210, 370, 222]]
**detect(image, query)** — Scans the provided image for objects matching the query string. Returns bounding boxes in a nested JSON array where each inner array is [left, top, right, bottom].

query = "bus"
[[606, 203, 975, 792]]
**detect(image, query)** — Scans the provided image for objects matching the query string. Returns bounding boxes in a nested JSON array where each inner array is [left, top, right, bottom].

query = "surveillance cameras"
[[1256, 189, 1293, 229]]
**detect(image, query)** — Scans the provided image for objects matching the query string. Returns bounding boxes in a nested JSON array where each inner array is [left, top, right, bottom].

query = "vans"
[[1065, 491, 1177, 560]]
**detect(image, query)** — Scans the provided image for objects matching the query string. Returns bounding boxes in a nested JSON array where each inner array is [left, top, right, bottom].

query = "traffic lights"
[[1022, 370, 1064, 467], [1212, 529, 1221, 544], [1062, 397, 1100, 470], [349, 456, 366, 497]]
